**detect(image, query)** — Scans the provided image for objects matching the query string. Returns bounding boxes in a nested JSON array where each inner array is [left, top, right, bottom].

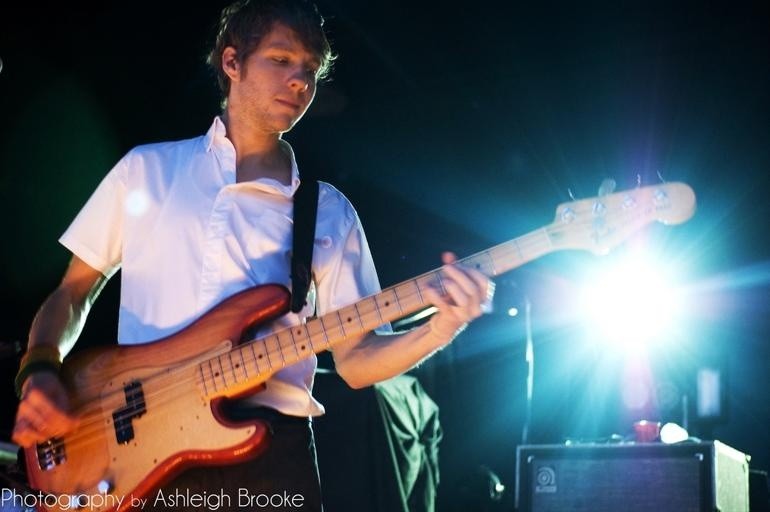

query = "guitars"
[[23, 170, 696, 512]]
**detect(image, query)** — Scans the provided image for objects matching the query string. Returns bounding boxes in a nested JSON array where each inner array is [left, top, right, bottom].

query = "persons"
[[6, 0, 497, 512]]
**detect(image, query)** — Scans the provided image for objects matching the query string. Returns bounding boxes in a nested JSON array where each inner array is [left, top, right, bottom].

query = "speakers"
[[515, 440, 752, 512]]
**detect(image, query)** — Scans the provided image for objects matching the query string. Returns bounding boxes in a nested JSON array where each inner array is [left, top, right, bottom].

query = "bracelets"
[[13, 340, 63, 400], [432, 317, 458, 339]]
